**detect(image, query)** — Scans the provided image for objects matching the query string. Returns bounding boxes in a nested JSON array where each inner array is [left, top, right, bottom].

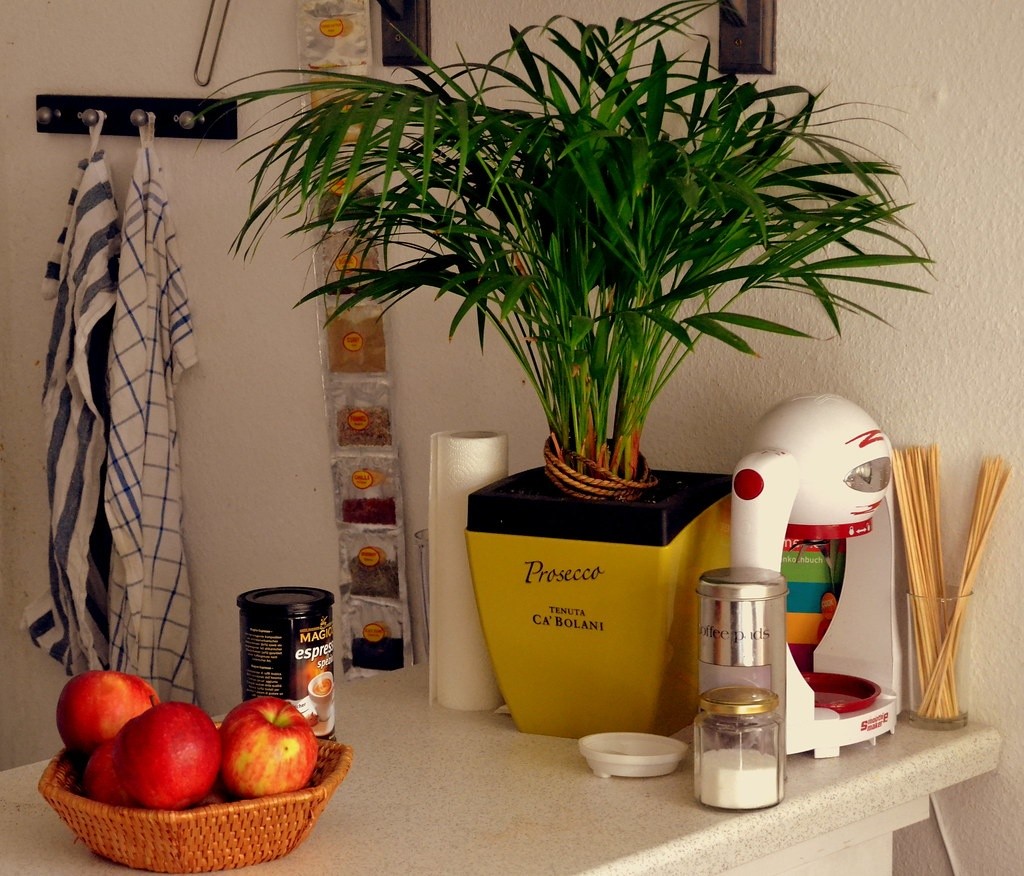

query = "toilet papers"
[[427, 431, 508, 709]]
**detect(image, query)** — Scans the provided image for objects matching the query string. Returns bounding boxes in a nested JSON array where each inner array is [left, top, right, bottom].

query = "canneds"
[[236, 587, 338, 742]]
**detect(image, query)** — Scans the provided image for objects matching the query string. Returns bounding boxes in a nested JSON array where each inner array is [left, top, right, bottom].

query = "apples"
[[57, 670, 318, 811]]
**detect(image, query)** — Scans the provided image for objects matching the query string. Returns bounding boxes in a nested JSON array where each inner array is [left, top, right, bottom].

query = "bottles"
[[694, 685, 785, 810]]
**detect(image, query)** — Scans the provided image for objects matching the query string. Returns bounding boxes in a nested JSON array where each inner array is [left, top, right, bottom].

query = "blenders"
[[731, 394, 902, 758]]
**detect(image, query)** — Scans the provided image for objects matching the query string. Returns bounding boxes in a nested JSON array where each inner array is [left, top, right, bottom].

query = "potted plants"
[[187, 0, 940, 727]]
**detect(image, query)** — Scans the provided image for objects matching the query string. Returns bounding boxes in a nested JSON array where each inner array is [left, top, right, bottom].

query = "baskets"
[[38, 718, 355, 876]]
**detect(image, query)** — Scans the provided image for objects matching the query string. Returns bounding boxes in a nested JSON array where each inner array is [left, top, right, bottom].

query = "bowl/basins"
[[578, 731, 689, 779]]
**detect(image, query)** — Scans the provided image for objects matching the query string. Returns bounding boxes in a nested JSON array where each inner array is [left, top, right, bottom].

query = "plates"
[[297, 695, 334, 735]]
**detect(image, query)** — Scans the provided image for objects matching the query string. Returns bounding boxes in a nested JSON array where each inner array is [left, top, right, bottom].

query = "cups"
[[906, 586, 973, 730], [695, 567, 791, 712], [307, 671, 334, 722]]
